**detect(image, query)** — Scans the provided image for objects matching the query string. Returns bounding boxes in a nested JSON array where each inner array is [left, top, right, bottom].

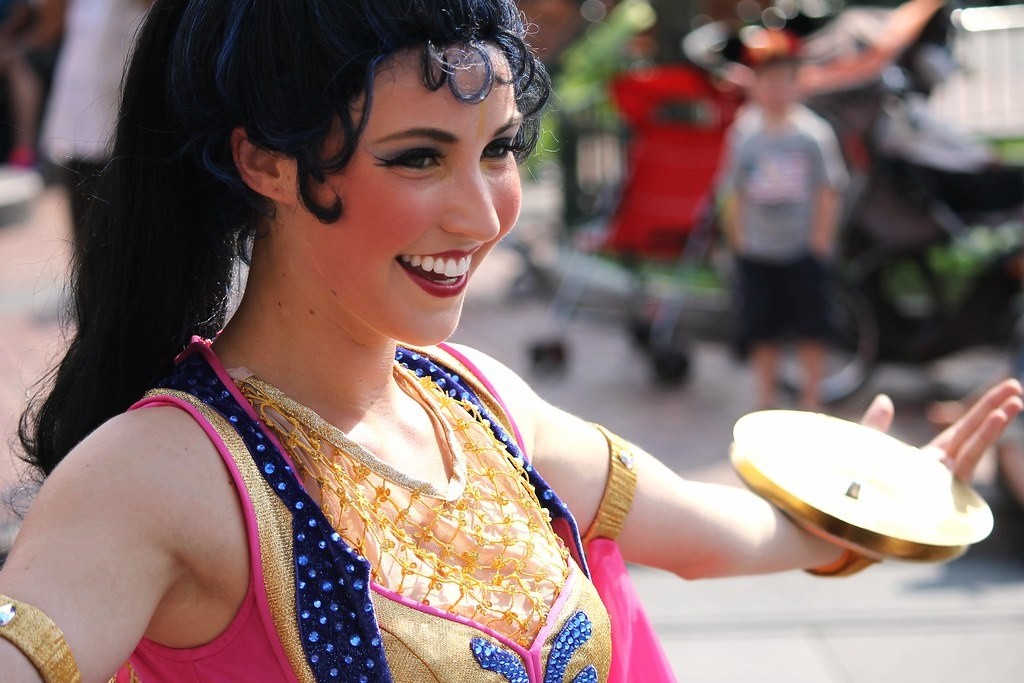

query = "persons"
[[46, 1, 158, 471], [1, 0, 1024, 683], [766, 0, 980, 409], [715, 25, 853, 424]]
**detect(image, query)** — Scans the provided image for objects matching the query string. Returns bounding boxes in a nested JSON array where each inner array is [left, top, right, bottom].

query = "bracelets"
[[806, 537, 884, 577]]
